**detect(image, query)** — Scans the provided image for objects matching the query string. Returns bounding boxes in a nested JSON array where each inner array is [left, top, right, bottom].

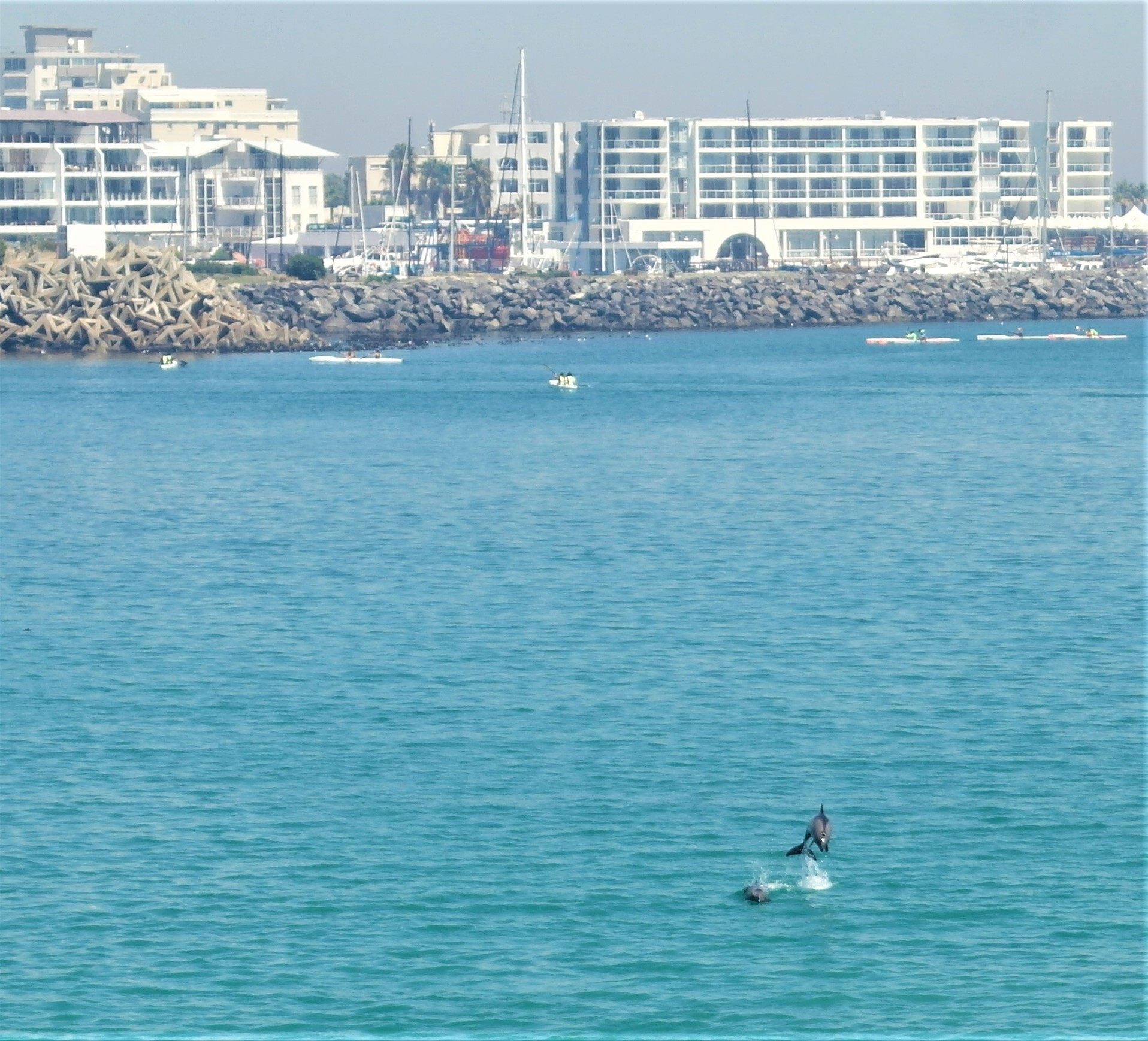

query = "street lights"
[[822, 231, 839, 261]]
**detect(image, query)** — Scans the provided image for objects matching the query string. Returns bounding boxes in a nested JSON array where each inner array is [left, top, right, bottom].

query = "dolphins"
[[786, 803, 832, 861], [744, 880, 768, 903]]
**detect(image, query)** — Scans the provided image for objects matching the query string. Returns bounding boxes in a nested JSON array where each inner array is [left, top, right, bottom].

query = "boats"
[[866, 337, 960, 343], [976, 334, 1127, 339], [549, 379, 577, 388], [309, 355, 403, 363], [160, 359, 177, 370]]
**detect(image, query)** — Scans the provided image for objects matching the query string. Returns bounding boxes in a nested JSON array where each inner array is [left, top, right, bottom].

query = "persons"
[[345, 347, 354, 358], [161, 353, 174, 364], [905, 330, 916, 339], [1011, 327, 1023, 336], [918, 329, 925, 340], [555, 372, 575, 384], [368, 349, 382, 358], [1087, 326, 1098, 336]]
